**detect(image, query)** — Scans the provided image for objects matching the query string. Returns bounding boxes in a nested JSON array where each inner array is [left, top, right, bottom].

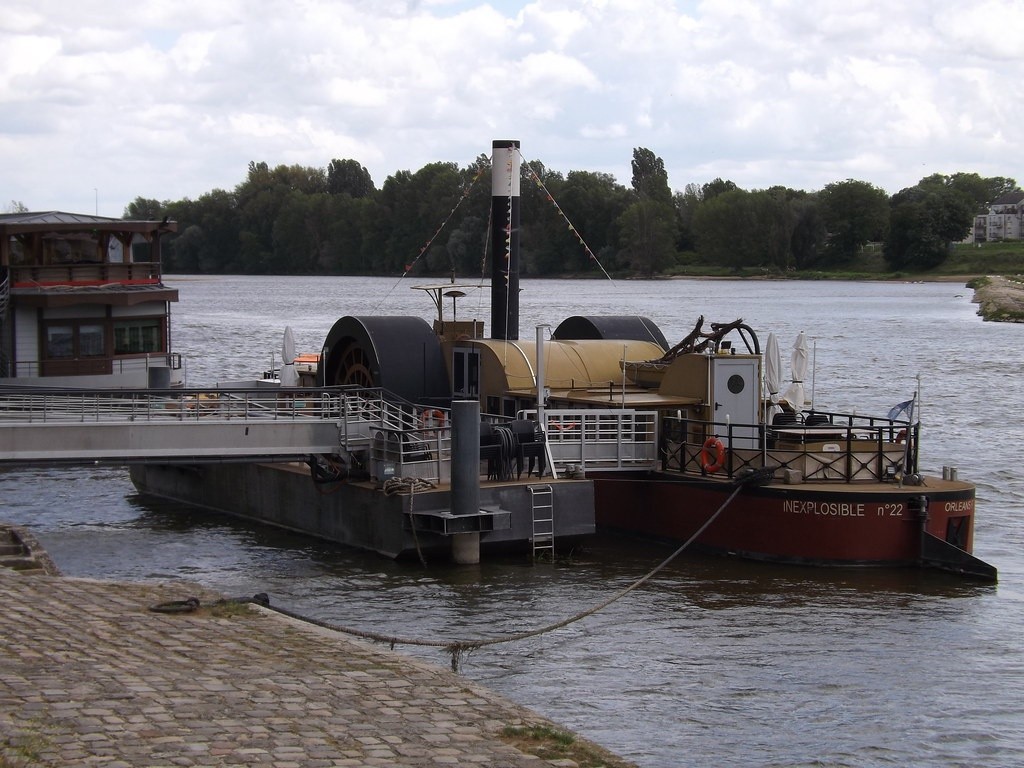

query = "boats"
[[1, 212, 185, 400]]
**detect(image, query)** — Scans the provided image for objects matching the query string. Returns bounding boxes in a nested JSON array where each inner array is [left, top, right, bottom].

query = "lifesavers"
[[700, 437, 725, 473], [418, 409, 446, 439]]
